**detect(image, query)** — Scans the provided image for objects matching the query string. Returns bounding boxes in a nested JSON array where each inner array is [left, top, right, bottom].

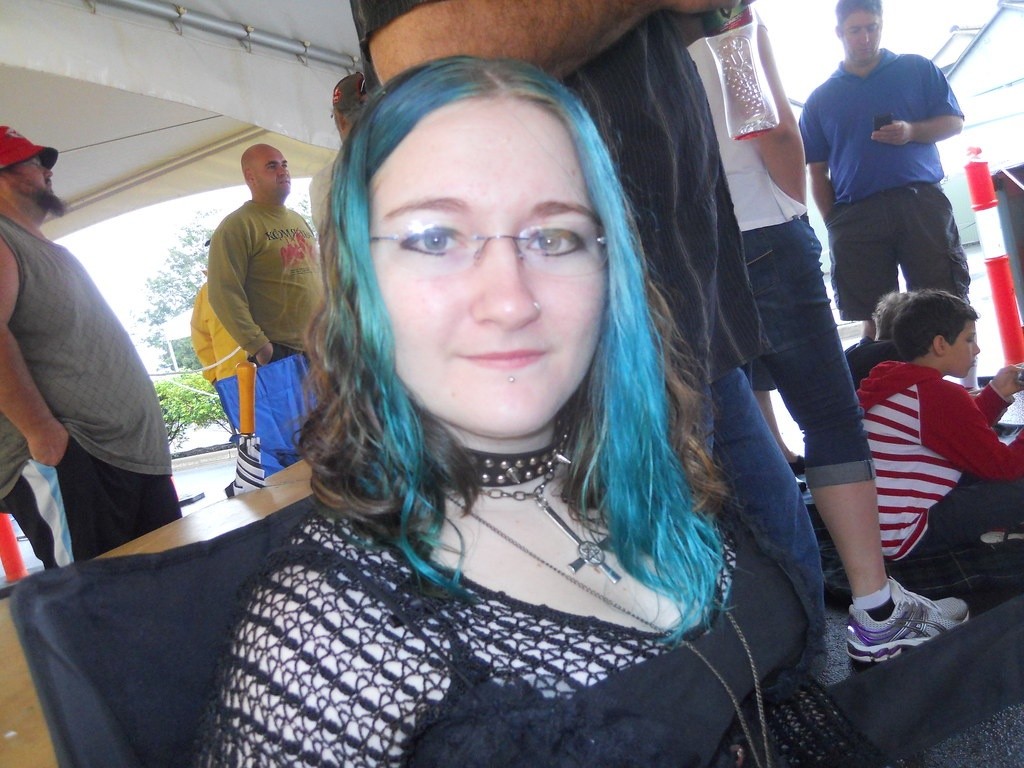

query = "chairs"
[[9, 488, 324, 768]]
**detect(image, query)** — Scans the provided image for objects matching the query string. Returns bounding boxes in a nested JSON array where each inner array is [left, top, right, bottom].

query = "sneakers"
[[845, 575, 970, 662]]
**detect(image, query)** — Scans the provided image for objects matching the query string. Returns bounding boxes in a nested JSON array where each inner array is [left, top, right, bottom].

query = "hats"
[[330, 71, 368, 119], [0, 125, 58, 170]]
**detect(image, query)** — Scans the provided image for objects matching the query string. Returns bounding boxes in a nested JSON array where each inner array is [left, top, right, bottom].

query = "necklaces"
[[424, 412, 772, 768]]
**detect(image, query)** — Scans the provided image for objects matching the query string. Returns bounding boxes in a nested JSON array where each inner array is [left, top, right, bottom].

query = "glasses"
[[368, 221, 612, 277]]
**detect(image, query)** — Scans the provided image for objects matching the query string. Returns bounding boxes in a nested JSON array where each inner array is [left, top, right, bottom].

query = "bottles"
[[700, 0, 779, 141]]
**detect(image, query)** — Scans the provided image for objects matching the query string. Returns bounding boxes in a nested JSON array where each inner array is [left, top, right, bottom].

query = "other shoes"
[[786, 455, 817, 504]]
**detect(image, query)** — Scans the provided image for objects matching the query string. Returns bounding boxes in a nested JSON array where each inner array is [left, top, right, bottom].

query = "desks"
[[0, 455, 317, 768]]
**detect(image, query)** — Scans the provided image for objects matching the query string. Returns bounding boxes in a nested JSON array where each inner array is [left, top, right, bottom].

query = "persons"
[[198, 50, 1024, 765], [0, 125, 182, 569], [204, 143, 325, 477], [686, 0, 971, 664], [190, 239, 248, 383], [351, 0, 827, 682], [840, 291, 1024, 564], [798, 0, 981, 396], [309, 69, 371, 240]]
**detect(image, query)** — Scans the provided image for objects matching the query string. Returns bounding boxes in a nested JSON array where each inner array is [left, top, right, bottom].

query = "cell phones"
[[873, 112, 893, 131]]
